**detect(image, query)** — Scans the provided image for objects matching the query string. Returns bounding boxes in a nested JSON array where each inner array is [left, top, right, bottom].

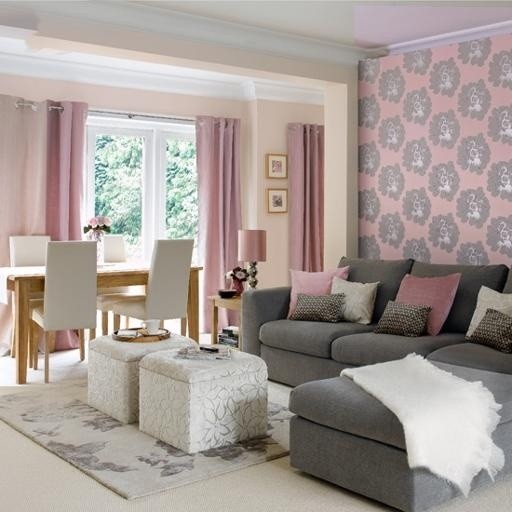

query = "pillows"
[[287, 265, 350, 318], [331, 276, 380, 325], [374, 299, 432, 337], [465, 285, 512, 341], [289, 293, 345, 322], [469, 308, 512, 353], [395, 273, 461, 336], [410, 261, 509, 333], [337, 256, 413, 320]]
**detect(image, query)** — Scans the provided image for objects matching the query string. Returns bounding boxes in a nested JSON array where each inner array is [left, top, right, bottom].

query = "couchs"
[[242, 285, 512, 512]]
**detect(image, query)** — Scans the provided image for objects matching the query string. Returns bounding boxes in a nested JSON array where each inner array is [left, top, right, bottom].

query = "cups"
[[141, 319, 161, 334]]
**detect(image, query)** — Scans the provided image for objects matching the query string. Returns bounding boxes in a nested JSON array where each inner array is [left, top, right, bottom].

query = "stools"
[[138, 345, 268, 455], [86, 329, 197, 425]]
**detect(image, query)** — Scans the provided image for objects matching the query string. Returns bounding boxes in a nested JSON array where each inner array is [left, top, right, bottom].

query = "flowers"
[[83, 216, 111, 234]]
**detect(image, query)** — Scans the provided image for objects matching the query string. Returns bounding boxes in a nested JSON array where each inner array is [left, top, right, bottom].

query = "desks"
[[237, 229, 267, 288], [0, 261, 203, 383]]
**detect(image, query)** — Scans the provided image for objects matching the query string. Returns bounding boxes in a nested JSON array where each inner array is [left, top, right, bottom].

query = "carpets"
[[0, 376, 295, 502]]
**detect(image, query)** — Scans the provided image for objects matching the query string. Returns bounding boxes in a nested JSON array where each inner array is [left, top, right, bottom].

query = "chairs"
[[30, 241, 97, 384], [9, 236, 56, 367], [114, 238, 194, 337], [97, 235, 127, 336]]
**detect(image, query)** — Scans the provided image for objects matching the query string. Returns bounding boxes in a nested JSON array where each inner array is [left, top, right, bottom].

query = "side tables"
[[208, 295, 242, 350]]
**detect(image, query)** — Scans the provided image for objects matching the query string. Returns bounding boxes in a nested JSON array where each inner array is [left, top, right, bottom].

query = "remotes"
[[199, 346, 219, 352]]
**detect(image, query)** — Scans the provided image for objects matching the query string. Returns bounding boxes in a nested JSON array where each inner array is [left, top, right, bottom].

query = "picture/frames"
[[266, 188, 288, 213], [265, 154, 288, 179]]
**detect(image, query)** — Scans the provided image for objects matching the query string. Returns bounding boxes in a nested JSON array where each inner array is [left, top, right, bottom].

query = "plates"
[[140, 329, 166, 336]]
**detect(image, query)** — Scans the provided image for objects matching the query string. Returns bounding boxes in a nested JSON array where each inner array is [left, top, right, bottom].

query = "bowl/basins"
[[218, 289, 238, 298]]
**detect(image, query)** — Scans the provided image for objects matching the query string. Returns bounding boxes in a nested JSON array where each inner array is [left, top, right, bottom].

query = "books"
[[176, 344, 232, 361]]
